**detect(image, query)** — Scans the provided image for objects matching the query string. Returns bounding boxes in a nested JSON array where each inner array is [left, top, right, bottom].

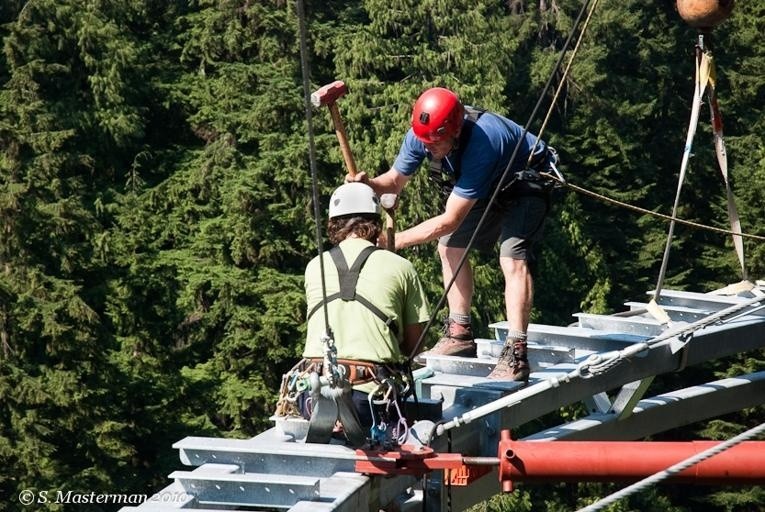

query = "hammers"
[[309, 80, 358, 177], [380, 192, 401, 254]]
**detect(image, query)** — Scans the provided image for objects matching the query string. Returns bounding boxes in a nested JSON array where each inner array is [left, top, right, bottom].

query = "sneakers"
[[416, 317, 477, 363], [486, 336, 530, 381]]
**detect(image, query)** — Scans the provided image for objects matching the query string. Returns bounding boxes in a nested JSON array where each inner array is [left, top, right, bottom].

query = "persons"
[[345, 87, 559, 383], [299, 182, 431, 439]]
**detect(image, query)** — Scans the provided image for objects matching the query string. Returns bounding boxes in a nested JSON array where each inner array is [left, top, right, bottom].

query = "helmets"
[[411, 87, 465, 144], [329, 182, 382, 221]]
